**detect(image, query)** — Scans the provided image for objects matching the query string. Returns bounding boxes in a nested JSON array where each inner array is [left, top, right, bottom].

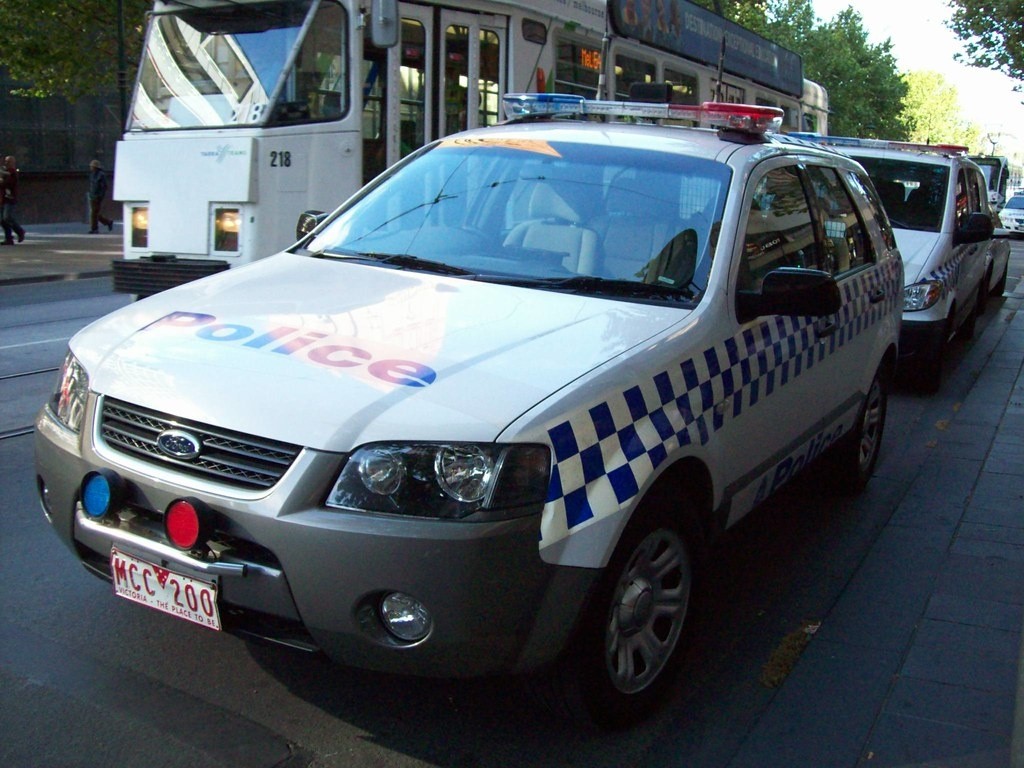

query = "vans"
[[969, 155, 1009, 213]]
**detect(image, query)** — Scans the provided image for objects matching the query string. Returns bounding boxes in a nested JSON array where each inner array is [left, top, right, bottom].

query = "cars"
[[986, 205, 1011, 299], [996, 194, 1024, 235], [32, 99, 910, 716], [778, 131, 993, 392]]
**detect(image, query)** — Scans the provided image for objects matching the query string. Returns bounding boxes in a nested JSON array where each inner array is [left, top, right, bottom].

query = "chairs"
[[905, 179, 940, 228], [592, 176, 680, 284], [870, 178, 904, 226], [500, 170, 602, 277]]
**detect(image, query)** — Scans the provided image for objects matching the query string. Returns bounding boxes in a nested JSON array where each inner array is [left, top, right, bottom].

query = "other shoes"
[[18, 229, 25, 242], [109, 220, 113, 231], [89, 229, 98, 233], [1, 236, 13, 245]]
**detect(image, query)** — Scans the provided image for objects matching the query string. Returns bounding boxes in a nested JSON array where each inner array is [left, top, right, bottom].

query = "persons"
[[88, 160, 113, 233], [0, 155, 25, 245]]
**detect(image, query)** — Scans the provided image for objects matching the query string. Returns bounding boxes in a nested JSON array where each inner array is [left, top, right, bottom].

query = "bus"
[[109, 0, 831, 303]]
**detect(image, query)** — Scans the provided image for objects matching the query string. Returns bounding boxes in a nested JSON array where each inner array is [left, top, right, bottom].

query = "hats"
[[90, 160, 100, 168]]
